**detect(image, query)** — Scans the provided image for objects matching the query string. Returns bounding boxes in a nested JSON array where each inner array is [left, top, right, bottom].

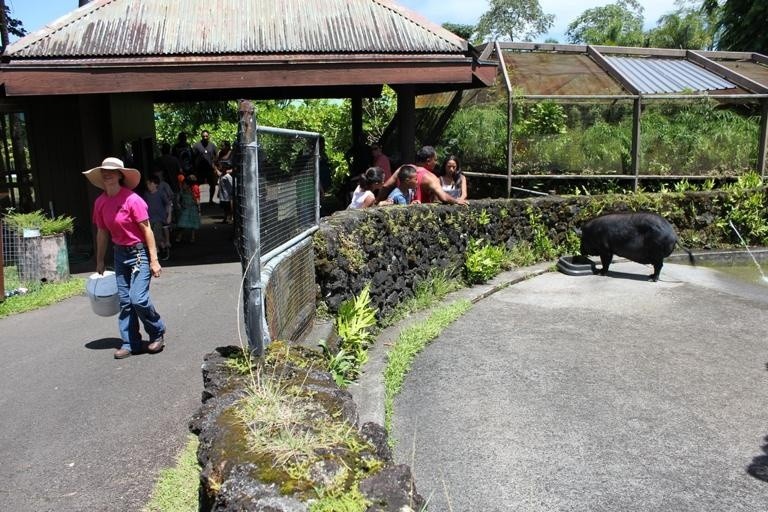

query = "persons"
[[141, 130, 237, 262], [293, 135, 330, 227], [342, 133, 470, 210], [82, 156, 165, 359]]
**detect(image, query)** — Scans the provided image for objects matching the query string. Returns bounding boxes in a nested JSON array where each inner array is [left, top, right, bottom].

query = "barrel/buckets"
[[86, 271, 121, 317]]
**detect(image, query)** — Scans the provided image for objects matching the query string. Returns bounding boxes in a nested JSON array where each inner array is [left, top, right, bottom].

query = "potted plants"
[[0, 207, 78, 286]]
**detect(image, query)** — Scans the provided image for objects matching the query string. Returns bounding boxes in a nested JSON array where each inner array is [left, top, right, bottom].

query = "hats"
[[81, 157, 141, 190]]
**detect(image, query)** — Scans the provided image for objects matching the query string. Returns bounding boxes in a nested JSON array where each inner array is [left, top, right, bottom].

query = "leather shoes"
[[147, 335, 164, 353], [114, 349, 131, 359]]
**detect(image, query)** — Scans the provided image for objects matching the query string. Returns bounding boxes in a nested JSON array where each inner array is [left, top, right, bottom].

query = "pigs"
[[573, 210, 696, 282]]
